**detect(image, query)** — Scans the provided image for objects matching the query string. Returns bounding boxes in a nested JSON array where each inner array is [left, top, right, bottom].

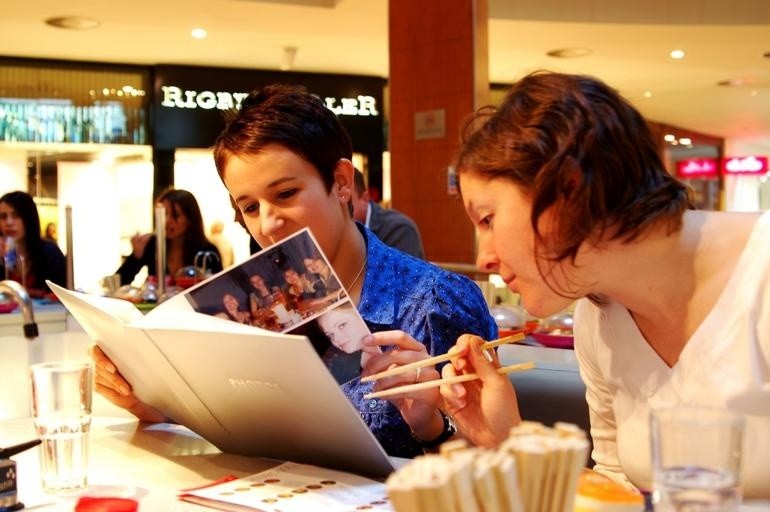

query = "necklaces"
[[345, 256, 368, 294]]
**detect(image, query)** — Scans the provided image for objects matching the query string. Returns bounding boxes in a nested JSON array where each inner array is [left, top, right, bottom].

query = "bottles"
[[2, 99, 148, 143], [4, 235, 27, 287]]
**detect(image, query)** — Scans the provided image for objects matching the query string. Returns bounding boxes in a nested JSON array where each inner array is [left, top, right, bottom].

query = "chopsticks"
[[359, 334, 536, 400], [382, 419, 592, 512]]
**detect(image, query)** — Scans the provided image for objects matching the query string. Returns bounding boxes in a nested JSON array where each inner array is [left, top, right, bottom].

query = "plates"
[[498, 314, 575, 348]]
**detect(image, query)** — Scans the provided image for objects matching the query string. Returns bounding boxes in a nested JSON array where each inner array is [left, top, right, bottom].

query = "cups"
[[30, 361, 95, 490], [648, 403, 747, 511]]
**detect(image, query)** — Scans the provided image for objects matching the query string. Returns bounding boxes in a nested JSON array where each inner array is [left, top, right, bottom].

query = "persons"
[[437, 72, 769, 510], [1, 190, 67, 305], [40, 222, 58, 247], [212, 76, 498, 460], [229, 166, 426, 265], [115, 187, 223, 295], [206, 252, 346, 327], [317, 297, 380, 387]]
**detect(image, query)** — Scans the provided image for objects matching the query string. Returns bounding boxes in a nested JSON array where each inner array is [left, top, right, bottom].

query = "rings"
[[412, 362, 420, 378]]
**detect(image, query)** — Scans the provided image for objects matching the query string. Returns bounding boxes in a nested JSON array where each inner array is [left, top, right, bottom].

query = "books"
[[45, 227, 397, 475]]
